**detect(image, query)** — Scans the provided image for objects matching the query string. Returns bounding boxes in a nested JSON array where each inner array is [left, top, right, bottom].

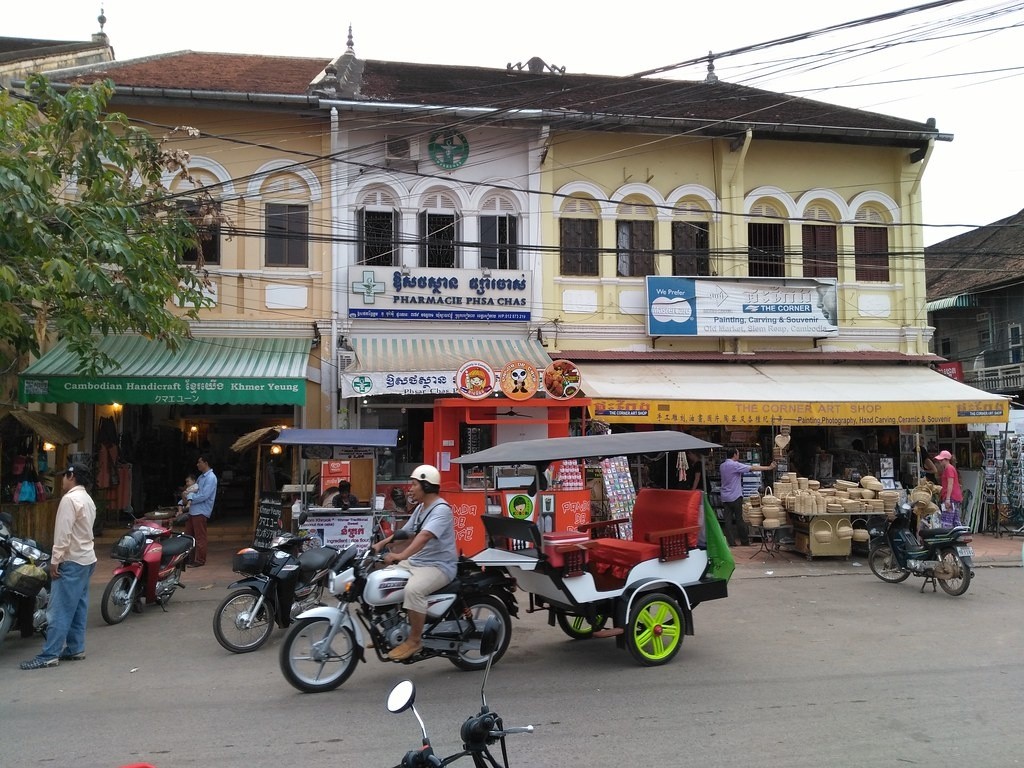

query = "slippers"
[[367, 641, 374, 648], [387, 642, 421, 659]]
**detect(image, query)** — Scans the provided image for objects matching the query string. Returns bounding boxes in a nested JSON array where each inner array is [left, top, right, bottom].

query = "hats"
[[934, 450, 952, 460], [56, 462, 91, 475]]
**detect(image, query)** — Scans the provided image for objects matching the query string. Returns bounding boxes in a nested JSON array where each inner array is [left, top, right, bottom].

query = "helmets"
[[409, 465, 440, 484]]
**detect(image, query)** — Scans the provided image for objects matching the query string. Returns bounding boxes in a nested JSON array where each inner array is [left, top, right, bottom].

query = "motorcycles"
[[101, 505, 195, 625], [0, 512, 52, 644], [868, 485, 975, 596], [386, 615, 534, 768], [213, 512, 340, 653], [279, 524, 520, 693]]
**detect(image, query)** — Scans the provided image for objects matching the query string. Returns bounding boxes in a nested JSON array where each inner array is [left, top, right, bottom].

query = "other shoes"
[[188, 562, 202, 568]]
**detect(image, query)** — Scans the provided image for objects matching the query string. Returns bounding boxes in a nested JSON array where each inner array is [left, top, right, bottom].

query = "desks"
[[748, 523, 794, 563], [374, 513, 412, 554]]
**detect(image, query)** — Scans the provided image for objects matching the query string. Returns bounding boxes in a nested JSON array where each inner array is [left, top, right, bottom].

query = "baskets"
[[111, 541, 143, 562], [6, 568, 44, 597], [911, 486, 937, 516], [851, 518, 869, 541], [762, 486, 776, 507], [812, 520, 833, 545], [835, 518, 853, 540], [233, 550, 267, 575]]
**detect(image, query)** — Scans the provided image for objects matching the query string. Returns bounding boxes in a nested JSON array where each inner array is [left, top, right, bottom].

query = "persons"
[[365, 465, 458, 659], [178, 456, 217, 567], [912, 446, 942, 512], [686, 450, 712, 502], [934, 451, 962, 526], [332, 481, 359, 507], [720, 450, 777, 546], [21, 463, 97, 669]]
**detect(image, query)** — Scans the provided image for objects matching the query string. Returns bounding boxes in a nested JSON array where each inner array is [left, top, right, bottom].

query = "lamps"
[[43, 442, 55, 451], [191, 423, 198, 432], [270, 444, 283, 455]]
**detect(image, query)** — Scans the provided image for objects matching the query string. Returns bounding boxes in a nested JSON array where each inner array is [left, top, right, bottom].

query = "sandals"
[[20, 655, 59, 670], [60, 652, 85, 661]]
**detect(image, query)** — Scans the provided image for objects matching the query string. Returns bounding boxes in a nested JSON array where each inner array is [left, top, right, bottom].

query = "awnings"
[[19, 336, 311, 405], [577, 365, 1010, 425], [341, 336, 555, 397]]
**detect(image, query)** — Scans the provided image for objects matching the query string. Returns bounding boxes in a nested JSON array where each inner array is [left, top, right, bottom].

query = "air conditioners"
[[338, 349, 358, 389], [385, 135, 419, 174]]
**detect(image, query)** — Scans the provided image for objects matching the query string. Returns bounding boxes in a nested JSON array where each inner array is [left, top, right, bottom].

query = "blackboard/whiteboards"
[[253, 497, 282, 551]]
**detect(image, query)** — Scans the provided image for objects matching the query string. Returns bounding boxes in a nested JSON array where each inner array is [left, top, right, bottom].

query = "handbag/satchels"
[[10, 556, 46, 594], [12, 437, 46, 504]]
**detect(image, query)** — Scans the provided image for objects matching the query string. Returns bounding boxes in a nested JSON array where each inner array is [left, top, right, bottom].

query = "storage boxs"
[[529, 531, 589, 568]]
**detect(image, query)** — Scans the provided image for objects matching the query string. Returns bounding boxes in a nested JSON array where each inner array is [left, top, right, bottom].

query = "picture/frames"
[[879, 423, 984, 532]]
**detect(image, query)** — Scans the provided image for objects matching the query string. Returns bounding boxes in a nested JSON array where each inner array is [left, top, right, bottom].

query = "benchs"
[[577, 488, 701, 580]]
